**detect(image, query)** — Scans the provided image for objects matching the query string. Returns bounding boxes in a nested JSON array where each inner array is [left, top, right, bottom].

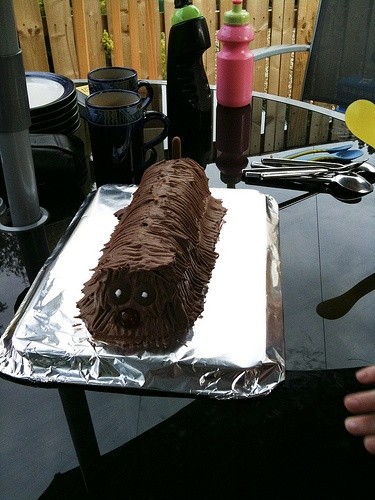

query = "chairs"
[[249, 1, 375, 107]]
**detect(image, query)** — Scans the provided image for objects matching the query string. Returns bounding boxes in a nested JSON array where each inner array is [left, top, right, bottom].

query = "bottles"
[[166, 1, 213, 113], [215, 0, 254, 108]]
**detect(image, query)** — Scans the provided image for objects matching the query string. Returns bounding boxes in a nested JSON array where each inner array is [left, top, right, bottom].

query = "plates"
[[25, 71, 81, 135]]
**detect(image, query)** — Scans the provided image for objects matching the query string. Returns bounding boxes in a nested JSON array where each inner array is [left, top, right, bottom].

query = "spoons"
[[242, 144, 373, 194]]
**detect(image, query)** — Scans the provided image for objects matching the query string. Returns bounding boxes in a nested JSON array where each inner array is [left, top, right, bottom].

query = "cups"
[[85, 67, 170, 177]]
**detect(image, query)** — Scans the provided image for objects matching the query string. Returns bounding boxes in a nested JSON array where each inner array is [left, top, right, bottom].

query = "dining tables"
[[0, 78, 375, 500]]
